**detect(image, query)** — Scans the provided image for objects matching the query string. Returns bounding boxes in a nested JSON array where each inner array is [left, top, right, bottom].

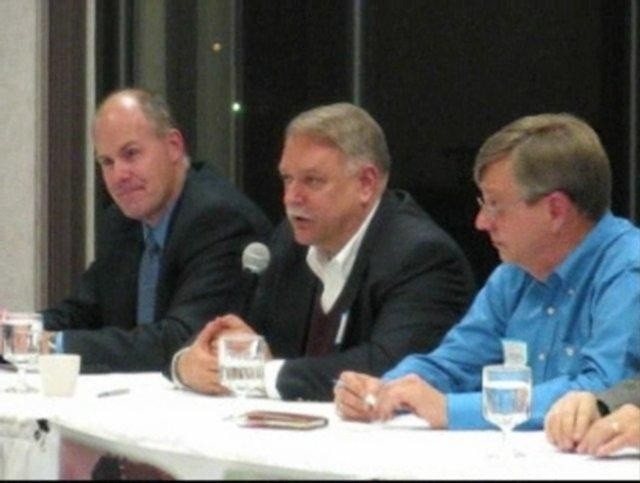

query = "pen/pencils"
[[334, 380, 377, 411], [99, 388, 129, 398]]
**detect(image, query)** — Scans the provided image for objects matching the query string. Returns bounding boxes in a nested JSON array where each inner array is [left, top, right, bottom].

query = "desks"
[[0, 368, 640, 482]]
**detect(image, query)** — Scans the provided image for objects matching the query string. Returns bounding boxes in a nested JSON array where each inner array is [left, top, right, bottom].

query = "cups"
[[35, 353, 79, 397]]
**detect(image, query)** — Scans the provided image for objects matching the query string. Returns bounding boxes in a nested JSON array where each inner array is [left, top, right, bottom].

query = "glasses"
[[477, 195, 522, 216]]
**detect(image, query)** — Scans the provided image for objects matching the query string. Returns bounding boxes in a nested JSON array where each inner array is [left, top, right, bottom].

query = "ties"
[[137, 233, 160, 326]]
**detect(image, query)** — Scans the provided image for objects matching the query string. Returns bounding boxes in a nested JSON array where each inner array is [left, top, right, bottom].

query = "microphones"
[[228, 241, 272, 316]]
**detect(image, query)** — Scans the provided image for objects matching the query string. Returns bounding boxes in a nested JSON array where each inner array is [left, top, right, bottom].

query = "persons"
[[542, 370, 640, 458], [333, 113, 640, 431], [0, 86, 275, 481], [160, 101, 477, 402]]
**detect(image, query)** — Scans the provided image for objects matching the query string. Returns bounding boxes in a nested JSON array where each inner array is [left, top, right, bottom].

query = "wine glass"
[[0, 314, 39, 401], [481, 363, 533, 460], [219, 336, 264, 425]]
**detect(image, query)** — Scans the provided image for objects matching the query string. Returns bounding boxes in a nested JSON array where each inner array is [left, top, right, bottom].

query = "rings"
[[613, 421, 618, 433]]
